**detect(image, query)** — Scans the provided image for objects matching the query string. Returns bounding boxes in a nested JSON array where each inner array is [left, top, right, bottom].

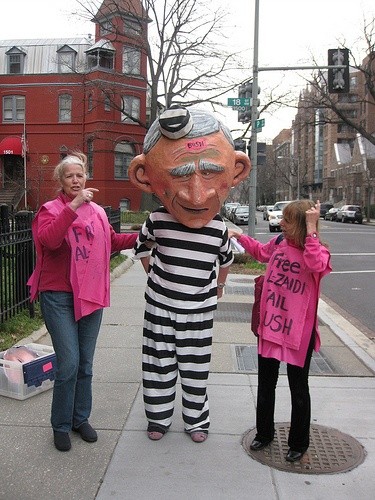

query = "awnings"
[[0, 136, 26, 155]]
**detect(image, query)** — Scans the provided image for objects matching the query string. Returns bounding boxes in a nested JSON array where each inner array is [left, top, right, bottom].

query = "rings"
[[88, 193, 92, 198]]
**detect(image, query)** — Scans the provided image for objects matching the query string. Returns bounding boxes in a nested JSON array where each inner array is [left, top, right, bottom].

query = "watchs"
[[217, 283, 225, 289], [306, 231, 319, 239]]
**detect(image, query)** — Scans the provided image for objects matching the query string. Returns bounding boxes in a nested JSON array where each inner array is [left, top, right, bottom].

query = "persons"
[[25, 157, 140, 449], [127, 103, 251, 438], [229, 199, 332, 463]]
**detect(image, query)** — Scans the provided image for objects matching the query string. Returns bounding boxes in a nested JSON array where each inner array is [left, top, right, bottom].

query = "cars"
[[222, 203, 241, 219], [257, 205, 274, 221], [229, 206, 257, 225], [324, 208, 340, 221]]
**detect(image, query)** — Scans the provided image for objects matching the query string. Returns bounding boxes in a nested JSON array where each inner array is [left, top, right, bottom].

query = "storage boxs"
[[0, 344, 58, 400]]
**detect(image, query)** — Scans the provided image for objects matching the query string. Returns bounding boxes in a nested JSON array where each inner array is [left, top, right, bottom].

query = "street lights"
[[277, 156, 300, 201]]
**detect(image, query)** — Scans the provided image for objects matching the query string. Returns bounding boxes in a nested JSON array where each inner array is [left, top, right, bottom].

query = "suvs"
[[320, 203, 333, 217], [336, 205, 363, 224], [268, 200, 293, 233]]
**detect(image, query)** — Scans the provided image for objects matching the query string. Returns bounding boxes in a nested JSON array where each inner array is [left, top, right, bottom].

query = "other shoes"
[[148, 431, 163, 441], [191, 432, 207, 443], [53, 429, 70, 452], [72, 423, 97, 443]]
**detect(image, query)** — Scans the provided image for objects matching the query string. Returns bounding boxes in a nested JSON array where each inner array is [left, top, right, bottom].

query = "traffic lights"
[[238, 83, 261, 123]]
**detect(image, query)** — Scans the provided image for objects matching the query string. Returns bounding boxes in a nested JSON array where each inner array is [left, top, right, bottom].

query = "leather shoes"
[[286, 448, 304, 462], [249, 439, 269, 450]]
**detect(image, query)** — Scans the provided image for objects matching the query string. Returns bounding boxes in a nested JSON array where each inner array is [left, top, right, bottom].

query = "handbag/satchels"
[[252, 275, 265, 336]]
